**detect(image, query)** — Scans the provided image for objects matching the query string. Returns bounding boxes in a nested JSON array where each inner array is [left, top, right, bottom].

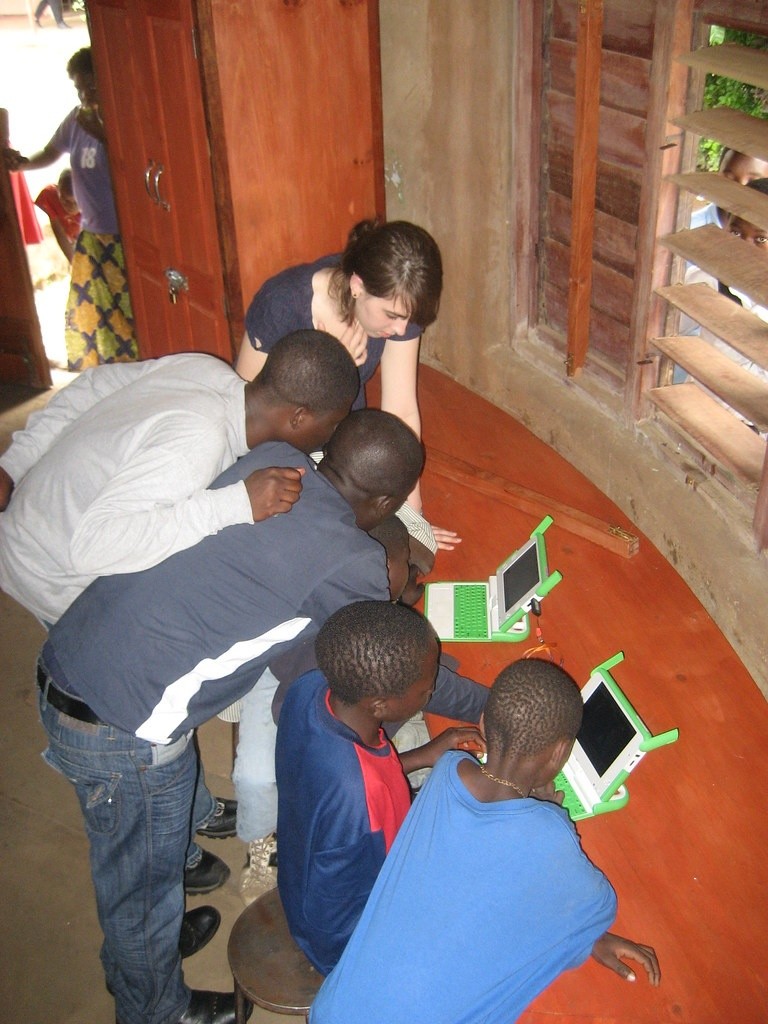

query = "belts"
[[36, 665, 110, 727]]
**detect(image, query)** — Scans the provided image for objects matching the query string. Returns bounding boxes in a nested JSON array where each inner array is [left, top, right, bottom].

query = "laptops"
[[425, 514, 564, 643], [479, 651, 681, 821]]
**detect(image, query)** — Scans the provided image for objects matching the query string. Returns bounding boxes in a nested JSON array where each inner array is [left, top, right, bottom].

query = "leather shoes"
[[179, 905, 222, 960], [177, 989, 254, 1024]]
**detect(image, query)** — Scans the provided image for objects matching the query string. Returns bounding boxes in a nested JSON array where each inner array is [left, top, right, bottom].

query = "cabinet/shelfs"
[[85, 0, 388, 370]]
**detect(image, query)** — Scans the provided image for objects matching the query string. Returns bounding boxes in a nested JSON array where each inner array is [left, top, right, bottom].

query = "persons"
[[276, 599, 487, 977], [37, 409, 423, 1024], [673, 146, 768, 385], [32, 0, 71, 29], [0, 329, 359, 896], [2, 46, 139, 373], [235, 220, 462, 551], [305, 658, 662, 1024], [34, 168, 82, 266], [679, 177, 768, 444]]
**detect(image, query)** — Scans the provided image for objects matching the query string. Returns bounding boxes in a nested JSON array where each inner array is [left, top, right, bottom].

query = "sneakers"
[[183, 850, 230, 895], [195, 797, 239, 839]]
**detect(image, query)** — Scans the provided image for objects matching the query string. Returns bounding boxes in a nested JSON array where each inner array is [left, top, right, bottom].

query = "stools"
[[226, 887, 326, 1024]]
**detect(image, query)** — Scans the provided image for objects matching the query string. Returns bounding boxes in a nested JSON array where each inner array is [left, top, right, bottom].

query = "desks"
[[366, 363, 768, 1024]]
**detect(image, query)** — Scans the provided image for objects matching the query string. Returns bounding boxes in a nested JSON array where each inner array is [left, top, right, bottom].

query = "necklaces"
[[479, 764, 526, 799]]
